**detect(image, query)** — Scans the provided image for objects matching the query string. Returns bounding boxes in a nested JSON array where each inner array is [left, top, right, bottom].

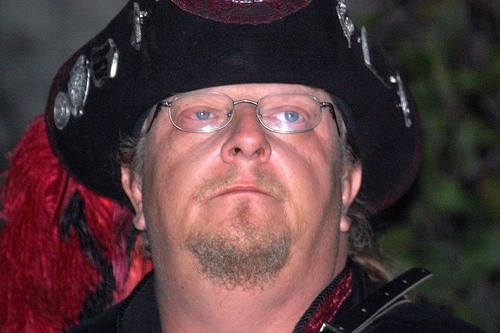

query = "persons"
[[44, 0, 486, 333]]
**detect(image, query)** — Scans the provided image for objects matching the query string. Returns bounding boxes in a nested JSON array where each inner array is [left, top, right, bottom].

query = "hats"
[[45, 0, 424, 217]]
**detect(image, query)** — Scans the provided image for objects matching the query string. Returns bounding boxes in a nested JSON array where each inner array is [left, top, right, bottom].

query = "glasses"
[[145, 90, 343, 136]]
